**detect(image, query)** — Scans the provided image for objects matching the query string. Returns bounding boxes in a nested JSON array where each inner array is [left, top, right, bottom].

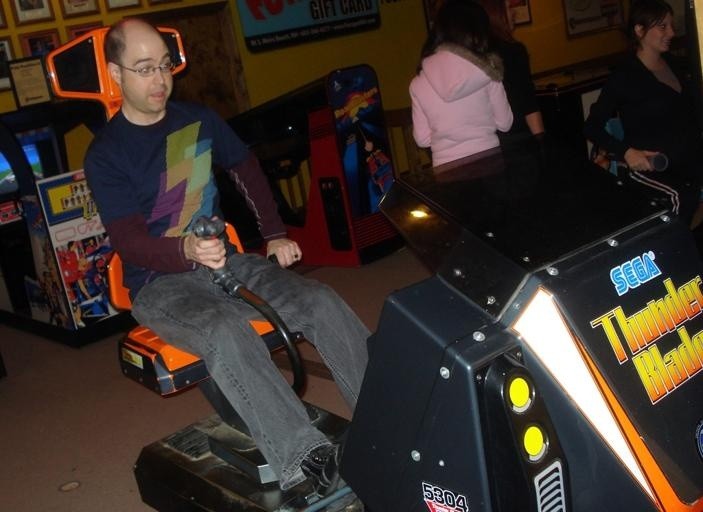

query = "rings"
[[293, 254, 299, 258]]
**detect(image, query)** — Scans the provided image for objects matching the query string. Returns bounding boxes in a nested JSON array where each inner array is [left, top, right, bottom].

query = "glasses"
[[115, 62, 175, 76]]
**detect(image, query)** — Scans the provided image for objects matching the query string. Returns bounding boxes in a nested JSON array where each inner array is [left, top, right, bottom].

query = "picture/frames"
[[560, 1, 623, 39], [1, 1, 142, 113]]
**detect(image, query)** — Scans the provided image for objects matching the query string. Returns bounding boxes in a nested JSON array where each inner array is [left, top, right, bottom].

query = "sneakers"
[[301, 448, 341, 496]]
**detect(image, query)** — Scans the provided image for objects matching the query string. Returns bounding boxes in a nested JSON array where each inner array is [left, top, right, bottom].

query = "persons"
[[409, 17, 514, 169], [417, 1, 545, 161], [581, 0, 703, 223], [80, 16, 374, 510]]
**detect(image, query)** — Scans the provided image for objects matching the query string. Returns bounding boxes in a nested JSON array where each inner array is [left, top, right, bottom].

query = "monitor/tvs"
[[0, 144, 46, 196]]
